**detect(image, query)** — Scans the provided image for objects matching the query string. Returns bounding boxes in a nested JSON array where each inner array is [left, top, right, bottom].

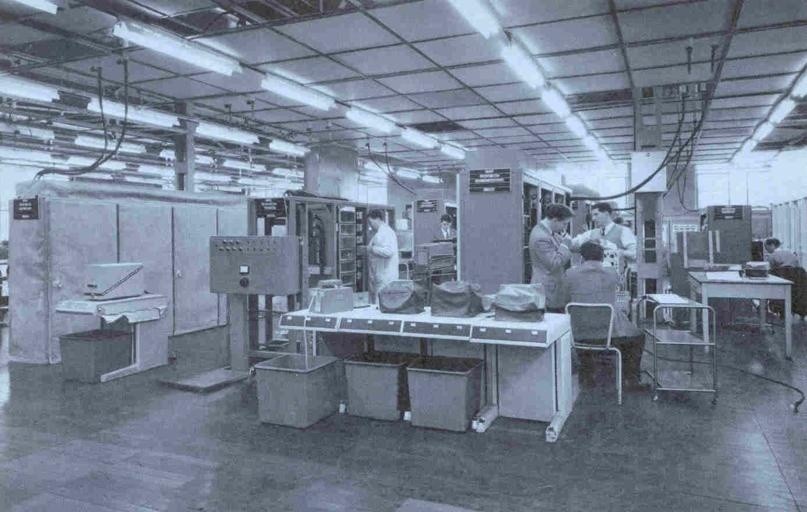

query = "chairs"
[[768, 267, 807, 320], [564, 302, 622, 405]]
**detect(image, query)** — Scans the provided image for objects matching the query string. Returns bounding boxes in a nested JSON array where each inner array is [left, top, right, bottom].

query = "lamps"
[[0, 0, 807, 190]]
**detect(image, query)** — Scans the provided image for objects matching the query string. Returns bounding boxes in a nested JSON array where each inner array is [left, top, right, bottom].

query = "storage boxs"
[[308, 287, 353, 313], [252, 355, 340, 428], [407, 355, 483, 432], [60, 328, 135, 385], [345, 350, 409, 421]]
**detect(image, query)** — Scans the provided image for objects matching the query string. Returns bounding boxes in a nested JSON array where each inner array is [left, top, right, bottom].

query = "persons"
[[431, 213, 456, 243], [364, 207, 399, 303], [529, 201, 652, 393], [763, 237, 800, 268]]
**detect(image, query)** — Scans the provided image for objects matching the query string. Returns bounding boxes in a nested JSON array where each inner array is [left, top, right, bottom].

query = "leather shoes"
[[622, 377, 650, 392]]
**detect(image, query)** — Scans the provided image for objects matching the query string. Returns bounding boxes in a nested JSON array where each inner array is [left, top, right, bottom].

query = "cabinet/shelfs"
[[215, 200, 251, 331], [303, 205, 394, 291], [10, 217, 51, 361], [39, 195, 117, 364], [117, 199, 174, 342], [172, 204, 218, 338], [463, 170, 571, 290]]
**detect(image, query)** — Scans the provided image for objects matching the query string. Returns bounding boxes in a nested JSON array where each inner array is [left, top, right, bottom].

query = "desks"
[[277, 303, 580, 443], [55, 295, 168, 383], [689, 271, 794, 361]]
